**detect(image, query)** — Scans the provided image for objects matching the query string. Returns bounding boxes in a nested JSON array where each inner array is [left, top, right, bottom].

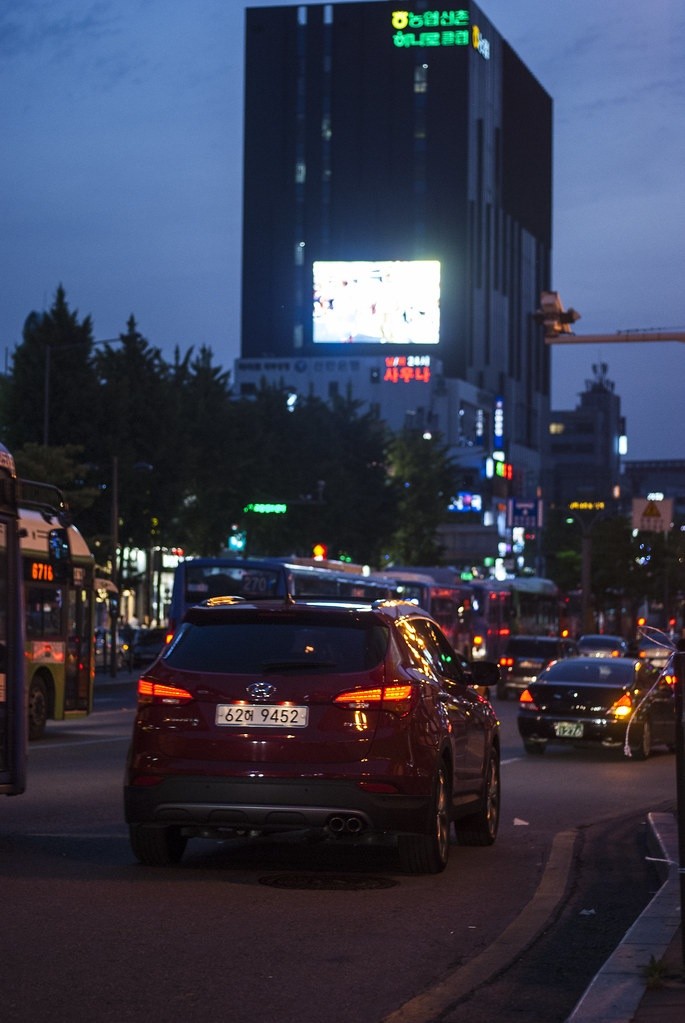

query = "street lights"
[[41, 330, 142, 445]]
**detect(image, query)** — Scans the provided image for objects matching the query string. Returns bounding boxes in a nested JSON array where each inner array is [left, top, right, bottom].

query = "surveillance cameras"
[[540, 291, 571, 334]]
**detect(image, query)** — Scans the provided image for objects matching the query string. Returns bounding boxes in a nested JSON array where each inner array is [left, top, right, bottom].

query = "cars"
[[573, 633, 629, 662], [634, 634, 678, 669], [517, 656, 679, 762], [658, 651, 684, 695]]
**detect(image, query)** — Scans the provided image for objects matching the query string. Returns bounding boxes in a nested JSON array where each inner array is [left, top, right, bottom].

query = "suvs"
[[496, 634, 578, 703], [123, 591, 501, 877]]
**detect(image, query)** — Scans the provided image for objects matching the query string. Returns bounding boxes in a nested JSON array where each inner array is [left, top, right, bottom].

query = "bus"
[[170, 555, 561, 680], [0, 474, 95, 738]]
